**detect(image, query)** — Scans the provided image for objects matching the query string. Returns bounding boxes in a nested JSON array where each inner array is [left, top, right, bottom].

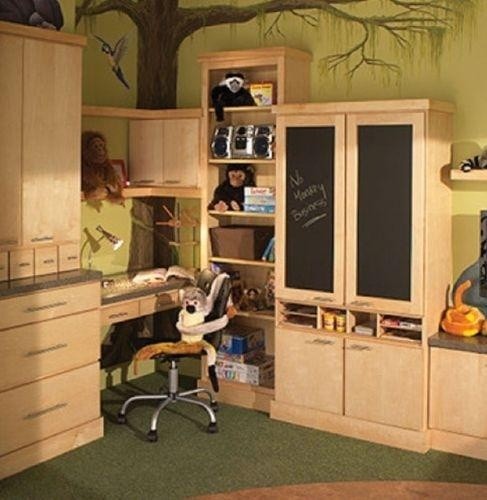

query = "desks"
[[100, 273, 195, 324]]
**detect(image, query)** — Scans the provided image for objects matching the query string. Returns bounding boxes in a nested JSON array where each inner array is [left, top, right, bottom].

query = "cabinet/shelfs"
[[427, 347, 486, 462], [195, 47, 313, 415]]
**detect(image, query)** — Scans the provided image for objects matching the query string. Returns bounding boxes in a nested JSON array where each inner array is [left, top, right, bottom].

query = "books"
[[130, 263, 192, 283]]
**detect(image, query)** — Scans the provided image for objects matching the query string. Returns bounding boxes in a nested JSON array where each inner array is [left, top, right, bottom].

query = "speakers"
[[253, 125, 274, 159], [211, 126, 234, 159]]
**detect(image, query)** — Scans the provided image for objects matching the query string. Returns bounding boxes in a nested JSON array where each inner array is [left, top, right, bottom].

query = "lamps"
[[88, 226, 124, 270]]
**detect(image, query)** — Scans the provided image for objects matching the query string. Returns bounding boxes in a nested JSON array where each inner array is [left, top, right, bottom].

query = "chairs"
[[118, 267, 234, 442]]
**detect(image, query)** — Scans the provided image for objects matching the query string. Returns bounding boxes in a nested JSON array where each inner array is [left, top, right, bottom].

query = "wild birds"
[[94, 32, 132, 91]]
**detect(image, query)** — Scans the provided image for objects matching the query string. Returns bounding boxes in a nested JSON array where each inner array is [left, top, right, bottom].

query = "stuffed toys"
[[438, 279, 486, 341], [209, 69, 257, 122], [226, 270, 274, 315], [134, 268, 238, 392], [80, 126, 123, 211], [206, 159, 258, 211]]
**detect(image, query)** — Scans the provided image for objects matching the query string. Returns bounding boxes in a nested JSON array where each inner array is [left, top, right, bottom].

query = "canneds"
[[323, 310, 345, 333]]
